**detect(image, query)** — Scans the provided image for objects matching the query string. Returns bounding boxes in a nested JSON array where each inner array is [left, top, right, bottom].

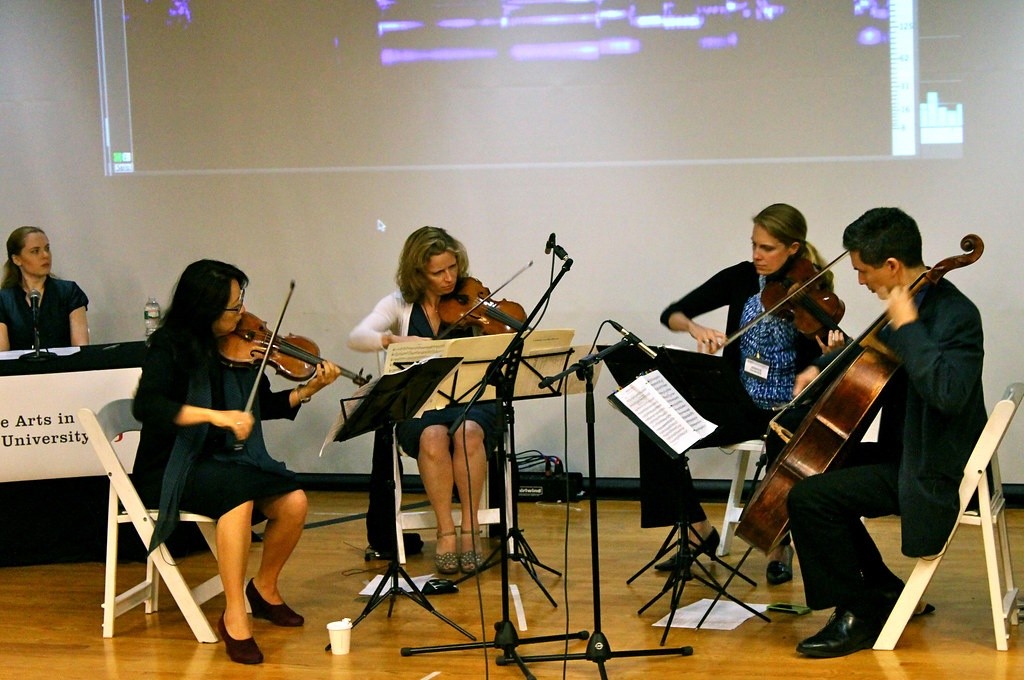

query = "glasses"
[[222, 288, 246, 312]]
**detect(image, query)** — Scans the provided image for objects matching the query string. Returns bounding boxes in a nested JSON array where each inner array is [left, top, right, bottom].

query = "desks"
[[0, 341, 210, 568]]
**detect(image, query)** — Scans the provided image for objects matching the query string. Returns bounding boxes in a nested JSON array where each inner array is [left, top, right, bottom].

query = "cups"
[[326, 621, 352, 655]]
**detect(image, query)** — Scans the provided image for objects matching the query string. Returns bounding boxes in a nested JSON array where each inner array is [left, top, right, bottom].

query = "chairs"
[[375, 346, 514, 564], [78, 399, 251, 643], [717, 440, 864, 557], [873, 383, 1024, 651]]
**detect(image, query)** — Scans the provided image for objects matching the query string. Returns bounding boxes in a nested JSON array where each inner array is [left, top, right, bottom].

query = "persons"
[[130, 258, 341, 664], [639, 203, 844, 586], [788, 207, 995, 661], [0, 226, 90, 352], [347, 227, 506, 574]]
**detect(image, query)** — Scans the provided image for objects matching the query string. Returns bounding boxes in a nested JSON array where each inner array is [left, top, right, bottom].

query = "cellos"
[[693, 234, 984, 631]]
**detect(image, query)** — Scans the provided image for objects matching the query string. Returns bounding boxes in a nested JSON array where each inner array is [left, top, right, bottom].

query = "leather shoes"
[[796, 595, 895, 655]]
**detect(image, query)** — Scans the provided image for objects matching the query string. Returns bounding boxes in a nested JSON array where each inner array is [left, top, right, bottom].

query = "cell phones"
[[767, 603, 811, 614]]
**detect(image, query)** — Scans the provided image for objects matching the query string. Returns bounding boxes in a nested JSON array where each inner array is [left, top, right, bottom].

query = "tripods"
[[323, 242, 771, 680]]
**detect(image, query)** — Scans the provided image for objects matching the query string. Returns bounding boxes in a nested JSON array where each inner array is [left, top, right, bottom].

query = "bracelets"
[[298, 383, 311, 404]]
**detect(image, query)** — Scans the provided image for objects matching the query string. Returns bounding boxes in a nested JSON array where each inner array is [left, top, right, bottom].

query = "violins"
[[437, 276, 534, 336], [214, 311, 372, 387], [759, 257, 855, 345]]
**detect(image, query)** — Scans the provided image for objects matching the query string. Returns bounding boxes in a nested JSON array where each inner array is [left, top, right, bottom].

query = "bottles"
[[144, 294, 160, 344]]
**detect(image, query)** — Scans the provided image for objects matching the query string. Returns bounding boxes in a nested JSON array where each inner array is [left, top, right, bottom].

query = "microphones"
[[545, 233, 555, 255], [609, 321, 657, 359], [28, 289, 41, 320]]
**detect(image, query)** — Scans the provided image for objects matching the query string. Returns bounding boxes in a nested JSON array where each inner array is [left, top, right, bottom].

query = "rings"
[[703, 339, 712, 344]]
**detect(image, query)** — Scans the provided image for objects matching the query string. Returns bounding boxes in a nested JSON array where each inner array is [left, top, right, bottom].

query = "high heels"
[[246, 577, 304, 627], [217, 609, 263, 664], [460, 528, 483, 573], [655, 526, 720, 570], [435, 531, 458, 574], [765, 546, 793, 585]]
